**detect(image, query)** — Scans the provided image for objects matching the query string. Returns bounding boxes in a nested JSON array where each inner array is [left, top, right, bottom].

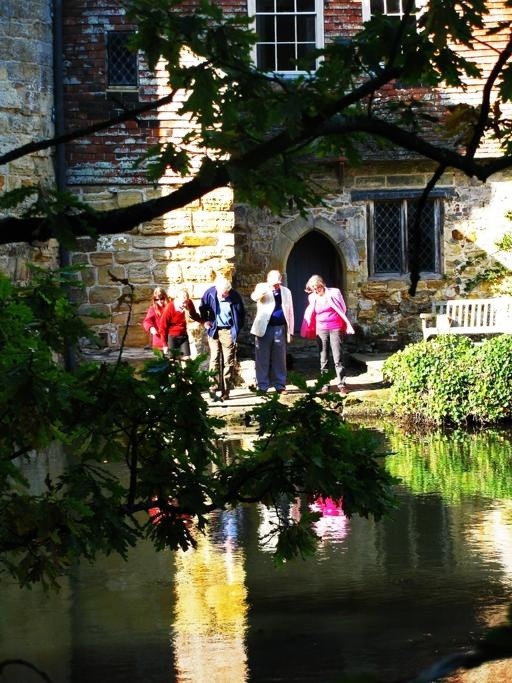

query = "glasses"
[[155, 298, 165, 301]]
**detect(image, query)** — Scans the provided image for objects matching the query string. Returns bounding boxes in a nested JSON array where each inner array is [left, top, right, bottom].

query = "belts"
[[217, 326, 231, 330]]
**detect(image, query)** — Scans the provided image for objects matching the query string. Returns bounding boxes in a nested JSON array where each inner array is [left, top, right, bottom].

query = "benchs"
[[420, 298, 510, 345]]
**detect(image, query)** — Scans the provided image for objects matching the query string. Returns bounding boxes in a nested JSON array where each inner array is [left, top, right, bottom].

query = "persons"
[[249, 269, 296, 397], [198, 276, 246, 401], [300, 275, 355, 396], [149, 495, 350, 550], [142, 286, 204, 358]]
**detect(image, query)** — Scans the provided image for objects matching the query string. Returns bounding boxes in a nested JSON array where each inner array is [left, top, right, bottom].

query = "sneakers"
[[277, 389, 288, 395], [257, 389, 266, 396], [209, 388, 216, 398], [337, 387, 348, 394], [221, 393, 229, 400], [322, 388, 328, 393]]
